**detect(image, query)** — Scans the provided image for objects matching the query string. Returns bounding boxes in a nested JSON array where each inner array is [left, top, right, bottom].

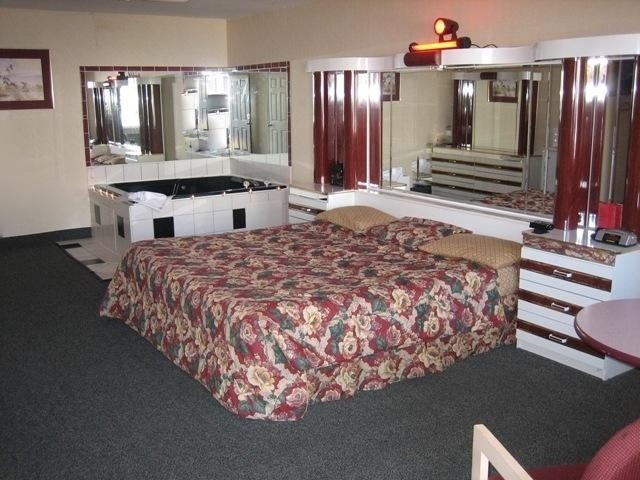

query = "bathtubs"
[[94, 174, 289, 204]]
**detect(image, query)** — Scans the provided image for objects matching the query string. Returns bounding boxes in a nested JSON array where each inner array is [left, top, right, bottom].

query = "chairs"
[[469, 408, 640, 479]]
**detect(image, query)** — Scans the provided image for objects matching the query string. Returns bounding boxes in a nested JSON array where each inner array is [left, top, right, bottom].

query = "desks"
[[574, 299, 640, 372]]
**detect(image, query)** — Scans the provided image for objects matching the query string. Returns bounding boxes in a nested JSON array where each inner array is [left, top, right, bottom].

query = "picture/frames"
[[382, 70, 401, 102], [0, 47, 55, 111], [487, 79, 519, 103]]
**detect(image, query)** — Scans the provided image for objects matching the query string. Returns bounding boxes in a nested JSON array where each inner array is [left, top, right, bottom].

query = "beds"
[[471, 189, 559, 218], [100, 205, 520, 429], [89, 143, 126, 165]]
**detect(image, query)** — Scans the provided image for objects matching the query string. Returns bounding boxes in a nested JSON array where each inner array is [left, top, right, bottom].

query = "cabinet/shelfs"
[[428, 145, 544, 204]]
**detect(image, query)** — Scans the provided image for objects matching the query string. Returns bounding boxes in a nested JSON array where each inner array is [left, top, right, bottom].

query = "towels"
[[128, 191, 169, 204]]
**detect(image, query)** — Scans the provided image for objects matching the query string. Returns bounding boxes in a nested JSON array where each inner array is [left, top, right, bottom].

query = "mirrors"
[[379, 63, 563, 224], [78, 59, 293, 167]]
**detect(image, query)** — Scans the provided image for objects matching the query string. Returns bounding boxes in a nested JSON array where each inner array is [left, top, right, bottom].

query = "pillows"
[[90, 143, 124, 164], [418, 231, 520, 271], [316, 203, 398, 237], [365, 212, 473, 254]]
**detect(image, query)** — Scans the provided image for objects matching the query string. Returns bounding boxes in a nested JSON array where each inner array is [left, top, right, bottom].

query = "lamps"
[[115, 70, 129, 88], [406, 16, 472, 55]]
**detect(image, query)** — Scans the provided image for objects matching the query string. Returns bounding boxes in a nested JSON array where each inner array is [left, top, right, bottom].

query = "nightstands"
[[515, 225, 639, 384], [288, 181, 367, 228]]
